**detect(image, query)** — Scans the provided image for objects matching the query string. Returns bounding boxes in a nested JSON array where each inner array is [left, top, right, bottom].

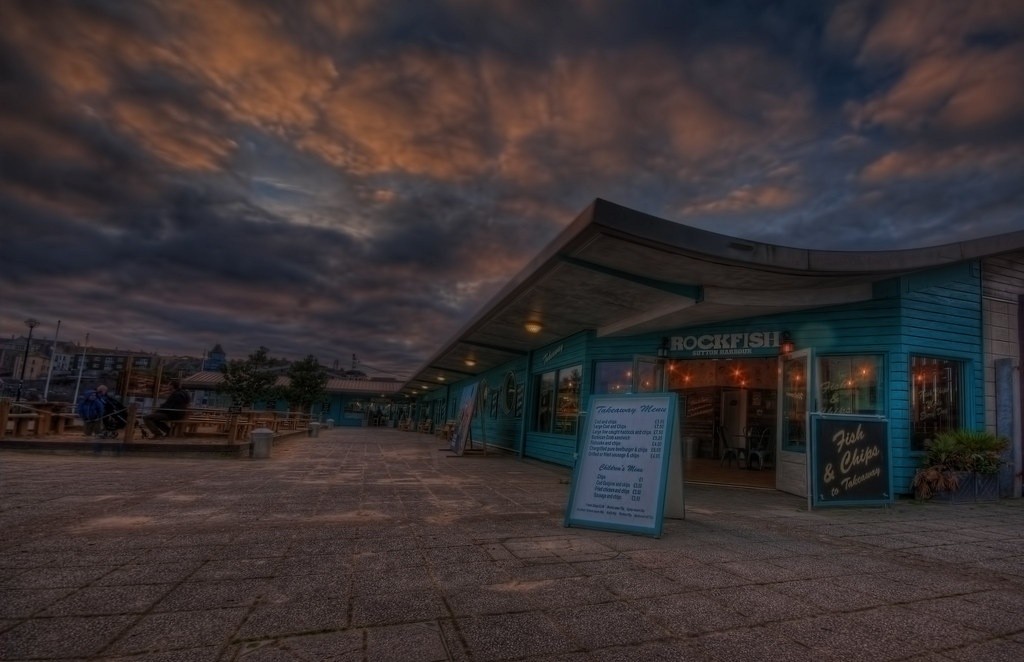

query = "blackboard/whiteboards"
[[810, 415, 894, 508], [563, 393, 684, 524]]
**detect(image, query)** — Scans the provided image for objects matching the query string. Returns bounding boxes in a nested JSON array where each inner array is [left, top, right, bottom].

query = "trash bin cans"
[[310, 422, 320, 437], [250, 428, 274, 459], [327, 419, 335, 430]]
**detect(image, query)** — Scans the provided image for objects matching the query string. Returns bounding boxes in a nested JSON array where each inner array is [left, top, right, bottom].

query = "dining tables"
[[734, 434, 761, 469]]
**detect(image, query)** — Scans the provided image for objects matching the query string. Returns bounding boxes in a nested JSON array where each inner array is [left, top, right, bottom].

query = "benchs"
[[5, 412, 80, 437]]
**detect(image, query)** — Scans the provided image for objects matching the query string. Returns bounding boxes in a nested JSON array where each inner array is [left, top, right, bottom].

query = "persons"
[[143, 379, 188, 440], [93, 385, 110, 408], [78, 390, 108, 438]]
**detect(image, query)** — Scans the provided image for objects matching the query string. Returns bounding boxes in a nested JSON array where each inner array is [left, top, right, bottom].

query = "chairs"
[[401, 417, 457, 440], [164, 414, 309, 441], [716, 425, 775, 473]]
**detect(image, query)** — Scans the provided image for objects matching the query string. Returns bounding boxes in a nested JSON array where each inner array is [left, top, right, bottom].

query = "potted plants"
[[909, 430, 1011, 504]]
